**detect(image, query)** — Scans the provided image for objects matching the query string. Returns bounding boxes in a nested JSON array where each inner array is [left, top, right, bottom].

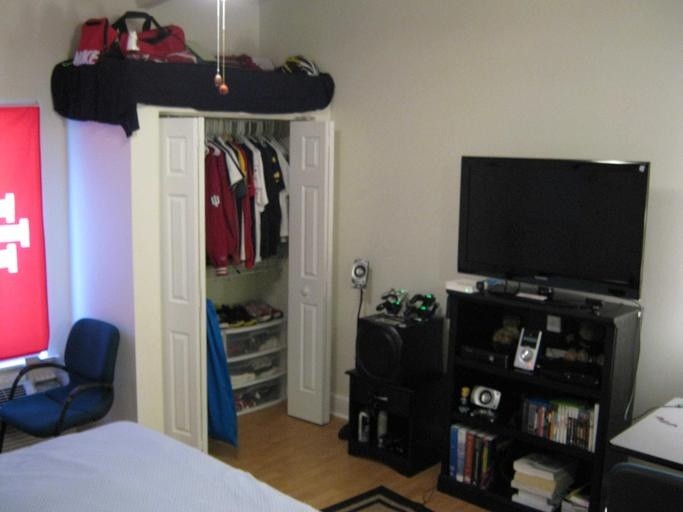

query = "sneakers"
[[258, 300, 285, 321], [213, 308, 230, 330], [244, 301, 272, 324], [231, 304, 258, 327], [222, 304, 245, 327]]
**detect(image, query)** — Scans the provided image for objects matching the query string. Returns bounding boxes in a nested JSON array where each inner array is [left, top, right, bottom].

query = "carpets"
[[318, 483, 434, 512]]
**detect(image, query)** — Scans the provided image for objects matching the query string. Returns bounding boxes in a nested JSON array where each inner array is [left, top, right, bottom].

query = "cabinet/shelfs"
[[433, 280, 647, 512], [343, 368, 449, 477], [67, 107, 336, 455], [216, 316, 287, 416]]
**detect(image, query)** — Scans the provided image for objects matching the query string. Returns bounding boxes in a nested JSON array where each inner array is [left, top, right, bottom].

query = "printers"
[[600, 394, 683, 512]]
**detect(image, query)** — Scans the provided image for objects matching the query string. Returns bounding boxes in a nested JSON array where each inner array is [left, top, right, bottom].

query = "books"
[[448, 395, 600, 512]]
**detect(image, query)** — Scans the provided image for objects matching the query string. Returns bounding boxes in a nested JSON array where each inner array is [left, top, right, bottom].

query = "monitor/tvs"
[[457, 155, 651, 310]]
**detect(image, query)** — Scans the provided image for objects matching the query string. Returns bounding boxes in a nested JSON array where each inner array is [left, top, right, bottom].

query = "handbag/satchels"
[[69, 9, 200, 67]]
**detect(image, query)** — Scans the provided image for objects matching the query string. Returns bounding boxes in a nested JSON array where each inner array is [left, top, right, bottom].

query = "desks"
[[607, 396, 682, 473]]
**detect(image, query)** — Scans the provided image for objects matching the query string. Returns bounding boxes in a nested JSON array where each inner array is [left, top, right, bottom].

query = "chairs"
[[0, 315, 124, 451], [595, 459, 681, 510]]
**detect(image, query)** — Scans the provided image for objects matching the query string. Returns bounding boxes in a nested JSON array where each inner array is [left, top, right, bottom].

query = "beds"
[[0, 421, 328, 512]]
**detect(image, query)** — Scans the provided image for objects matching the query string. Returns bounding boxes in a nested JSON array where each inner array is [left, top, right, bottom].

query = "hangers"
[[198, 115, 280, 154]]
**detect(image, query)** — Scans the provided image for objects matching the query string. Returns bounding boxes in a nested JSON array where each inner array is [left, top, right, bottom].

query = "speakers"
[[448, 370, 521, 434], [356, 313, 443, 389]]
